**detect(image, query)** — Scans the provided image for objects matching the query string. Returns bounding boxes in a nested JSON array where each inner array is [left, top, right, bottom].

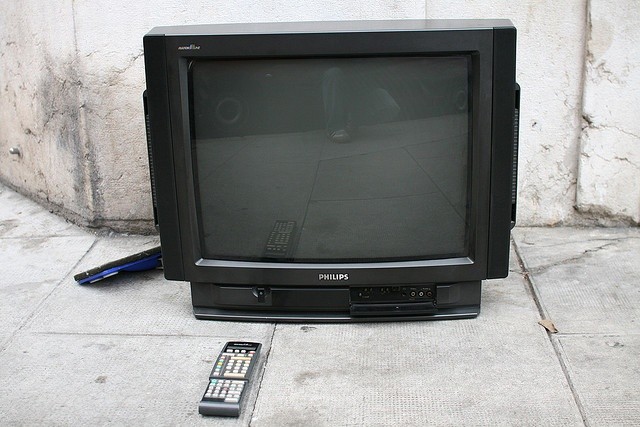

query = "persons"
[[322, 58, 401, 143]]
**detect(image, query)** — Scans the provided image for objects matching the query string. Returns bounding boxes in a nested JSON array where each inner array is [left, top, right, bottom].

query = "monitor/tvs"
[[143, 19, 520, 323]]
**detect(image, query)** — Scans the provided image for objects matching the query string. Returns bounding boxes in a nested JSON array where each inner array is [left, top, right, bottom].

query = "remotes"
[[199, 341, 262, 417], [262, 220, 297, 258]]
